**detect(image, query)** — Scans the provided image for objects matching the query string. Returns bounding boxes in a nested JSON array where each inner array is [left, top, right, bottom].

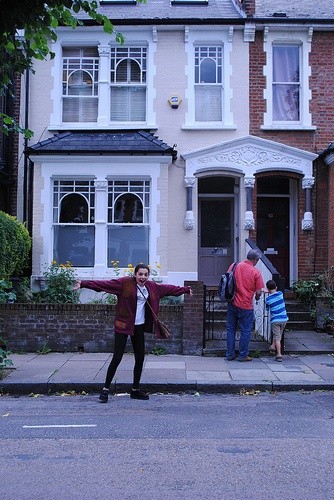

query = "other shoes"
[[269, 345, 275, 351], [276, 355, 283, 361]]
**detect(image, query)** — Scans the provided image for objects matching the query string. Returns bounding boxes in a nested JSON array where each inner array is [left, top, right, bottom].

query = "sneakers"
[[242, 356, 252, 361], [131, 389, 149, 400], [227, 357, 235, 361], [99, 390, 109, 401]]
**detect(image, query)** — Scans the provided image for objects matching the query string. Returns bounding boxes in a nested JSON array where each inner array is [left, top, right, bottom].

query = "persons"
[[264, 279, 289, 362], [225, 249, 265, 362], [72, 264, 193, 402]]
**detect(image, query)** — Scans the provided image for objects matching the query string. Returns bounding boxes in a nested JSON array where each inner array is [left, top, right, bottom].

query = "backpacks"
[[218, 261, 239, 304]]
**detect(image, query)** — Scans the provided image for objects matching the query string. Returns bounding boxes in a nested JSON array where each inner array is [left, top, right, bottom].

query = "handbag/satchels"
[[158, 321, 171, 340]]
